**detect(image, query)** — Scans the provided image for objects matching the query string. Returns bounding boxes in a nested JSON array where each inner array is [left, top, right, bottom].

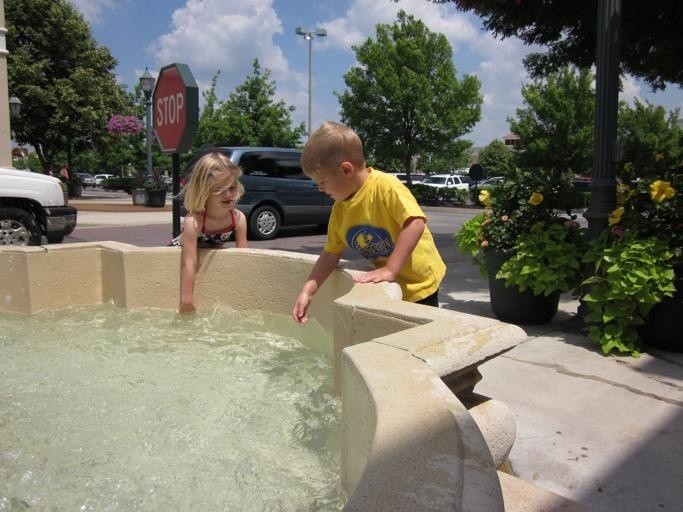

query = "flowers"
[[583, 178, 682, 356], [454, 174, 582, 296]]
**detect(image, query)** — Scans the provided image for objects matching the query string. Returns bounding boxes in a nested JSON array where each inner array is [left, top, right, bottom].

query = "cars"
[[0, 166, 77, 245], [415, 167, 507, 202]]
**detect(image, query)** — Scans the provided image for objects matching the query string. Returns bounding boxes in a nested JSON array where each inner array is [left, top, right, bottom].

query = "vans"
[[71, 172, 113, 189], [389, 173, 427, 186], [179, 147, 336, 241]]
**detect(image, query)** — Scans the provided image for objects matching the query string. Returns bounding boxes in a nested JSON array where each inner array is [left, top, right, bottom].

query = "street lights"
[[138, 67, 156, 175], [294, 26, 326, 140]]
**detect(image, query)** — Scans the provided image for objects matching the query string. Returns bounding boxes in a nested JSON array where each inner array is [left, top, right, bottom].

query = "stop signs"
[[149, 63, 198, 155]]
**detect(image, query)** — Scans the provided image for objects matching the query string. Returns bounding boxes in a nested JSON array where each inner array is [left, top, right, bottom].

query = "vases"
[[487, 244, 561, 325], [636, 277, 681, 355]]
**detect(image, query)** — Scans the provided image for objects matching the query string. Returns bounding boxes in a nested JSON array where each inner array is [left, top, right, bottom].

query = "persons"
[[47, 162, 70, 206], [290, 119, 448, 324], [168, 150, 248, 315]]
